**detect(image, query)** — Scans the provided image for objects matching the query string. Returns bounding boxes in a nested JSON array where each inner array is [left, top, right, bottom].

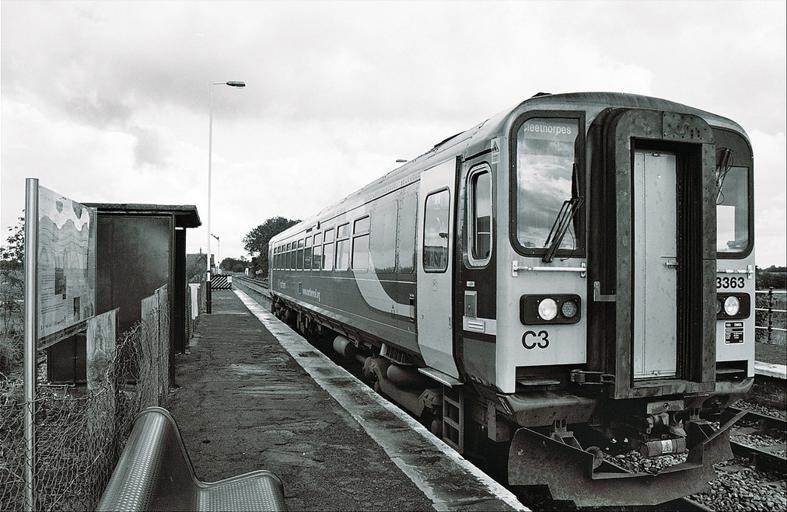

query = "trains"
[[268, 91, 755, 507]]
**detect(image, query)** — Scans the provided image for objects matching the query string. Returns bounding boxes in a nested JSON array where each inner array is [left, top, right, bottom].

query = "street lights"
[[207, 80, 245, 315]]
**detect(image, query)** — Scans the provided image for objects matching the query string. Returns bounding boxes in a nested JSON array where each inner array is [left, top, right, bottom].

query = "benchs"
[[94, 406, 286, 511]]
